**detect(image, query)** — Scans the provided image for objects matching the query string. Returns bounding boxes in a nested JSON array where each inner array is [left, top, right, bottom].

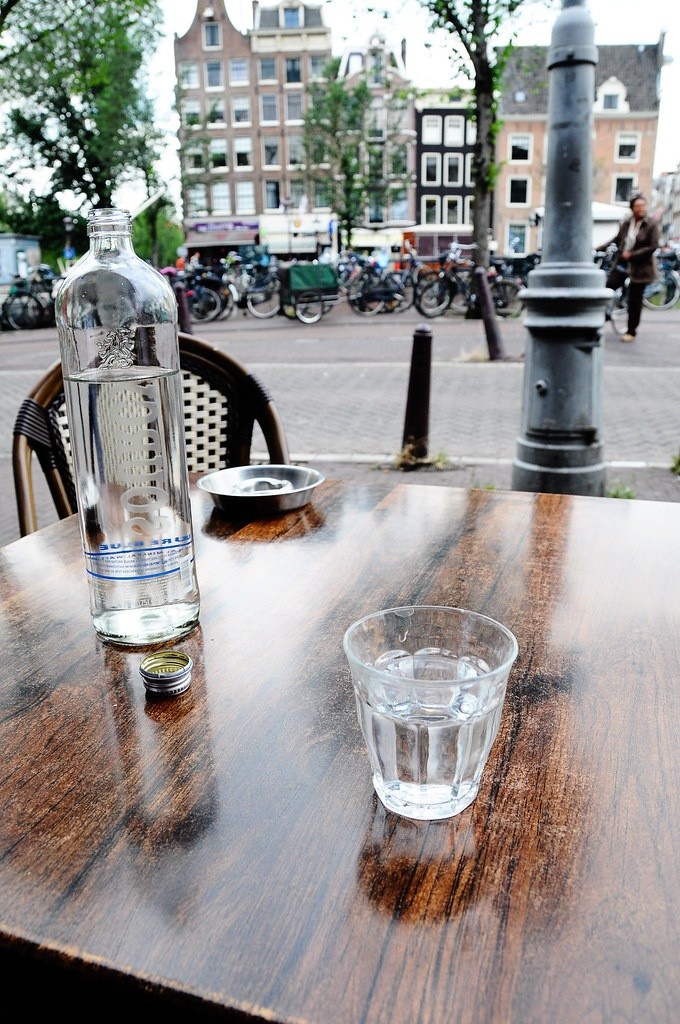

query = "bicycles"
[[1, 218, 680, 334]]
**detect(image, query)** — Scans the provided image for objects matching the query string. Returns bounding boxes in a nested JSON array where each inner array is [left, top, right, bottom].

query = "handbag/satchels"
[[606, 254, 633, 291]]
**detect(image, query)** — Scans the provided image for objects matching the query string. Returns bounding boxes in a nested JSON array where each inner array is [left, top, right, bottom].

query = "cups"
[[342, 605, 519, 820]]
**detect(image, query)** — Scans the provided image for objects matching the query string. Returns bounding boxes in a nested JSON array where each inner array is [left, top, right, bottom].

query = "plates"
[[196, 465, 326, 511]]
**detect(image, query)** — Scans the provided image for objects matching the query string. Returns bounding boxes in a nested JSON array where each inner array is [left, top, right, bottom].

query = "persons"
[[175, 236, 412, 305], [603, 195, 660, 341]]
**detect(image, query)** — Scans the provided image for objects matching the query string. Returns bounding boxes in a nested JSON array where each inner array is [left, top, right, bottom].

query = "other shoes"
[[621, 334, 635, 342]]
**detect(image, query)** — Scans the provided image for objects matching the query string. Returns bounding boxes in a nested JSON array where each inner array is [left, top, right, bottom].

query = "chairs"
[[12, 330, 287, 538]]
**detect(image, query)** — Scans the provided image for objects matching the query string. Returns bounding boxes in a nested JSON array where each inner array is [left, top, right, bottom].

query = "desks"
[[3, 460, 676, 1024]]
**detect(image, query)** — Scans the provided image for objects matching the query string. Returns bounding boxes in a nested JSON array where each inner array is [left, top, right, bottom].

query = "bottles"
[[55, 208, 201, 647]]
